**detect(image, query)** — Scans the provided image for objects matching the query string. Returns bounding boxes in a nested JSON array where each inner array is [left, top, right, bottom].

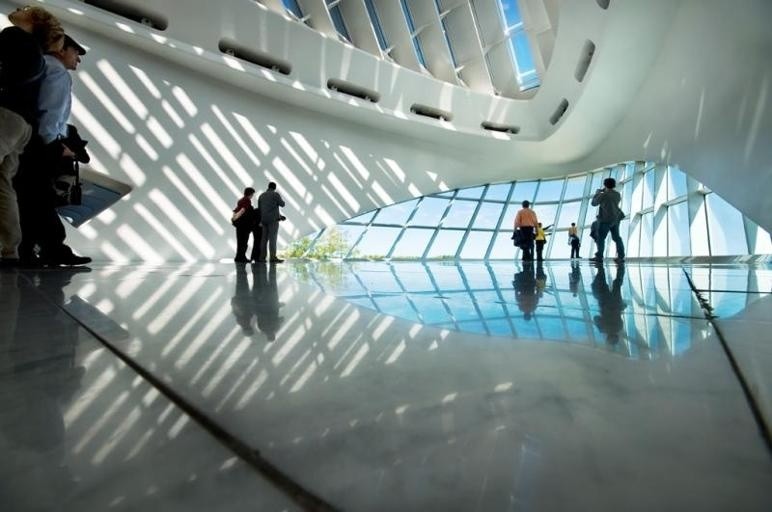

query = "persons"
[[510, 262, 550, 321], [0, 4, 92, 267], [231, 182, 286, 263], [568, 223, 582, 258], [589, 178, 626, 263], [589, 265, 628, 347], [230, 265, 285, 343], [512, 200, 554, 261], [567, 263, 582, 301], [3, 273, 86, 454]]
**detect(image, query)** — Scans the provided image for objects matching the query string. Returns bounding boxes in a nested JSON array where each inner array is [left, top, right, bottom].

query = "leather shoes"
[[19, 243, 90, 266], [589, 256, 604, 262], [235, 256, 284, 262], [615, 257, 625, 264]]
